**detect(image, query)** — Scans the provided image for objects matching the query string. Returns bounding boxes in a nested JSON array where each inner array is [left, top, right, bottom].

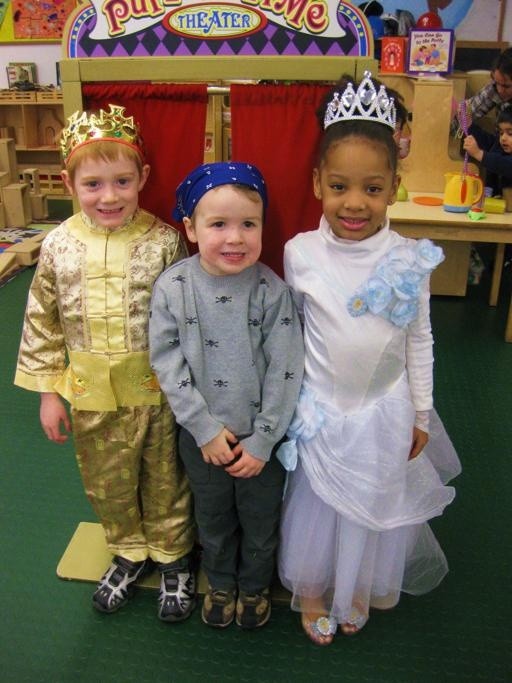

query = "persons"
[[147, 160, 305, 631], [13, 104, 197, 624], [463, 104, 512, 268], [276, 68, 463, 645], [450, 48, 512, 138], [411, 44, 441, 66]]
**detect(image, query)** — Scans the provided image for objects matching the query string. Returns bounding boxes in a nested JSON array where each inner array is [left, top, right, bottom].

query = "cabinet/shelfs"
[[1, 101, 66, 199], [379, 65, 501, 298]]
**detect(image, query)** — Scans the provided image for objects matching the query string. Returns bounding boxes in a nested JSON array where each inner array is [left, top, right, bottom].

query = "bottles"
[[396, 184, 409, 202]]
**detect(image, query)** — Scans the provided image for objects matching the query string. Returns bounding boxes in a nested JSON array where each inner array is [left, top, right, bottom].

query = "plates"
[[412, 196, 443, 207]]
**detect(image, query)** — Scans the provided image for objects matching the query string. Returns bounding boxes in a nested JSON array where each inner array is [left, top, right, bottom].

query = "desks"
[[378, 187, 511, 340]]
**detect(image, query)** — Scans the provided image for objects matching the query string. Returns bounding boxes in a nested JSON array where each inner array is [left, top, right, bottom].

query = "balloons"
[[416, 11, 442, 28]]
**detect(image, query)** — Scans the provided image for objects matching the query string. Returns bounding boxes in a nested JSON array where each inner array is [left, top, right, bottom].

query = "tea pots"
[[442, 171, 482, 212]]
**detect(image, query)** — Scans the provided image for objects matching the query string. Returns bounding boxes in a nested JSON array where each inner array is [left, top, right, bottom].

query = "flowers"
[[348, 237, 447, 331], [284, 384, 326, 440]]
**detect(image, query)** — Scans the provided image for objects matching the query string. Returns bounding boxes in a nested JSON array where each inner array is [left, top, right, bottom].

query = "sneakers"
[[236, 589, 271, 629], [155, 559, 197, 622], [91, 556, 147, 614], [202, 584, 236, 627]]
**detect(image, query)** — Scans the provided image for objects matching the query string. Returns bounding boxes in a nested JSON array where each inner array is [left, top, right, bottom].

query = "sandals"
[[339, 603, 367, 634], [300, 595, 337, 645]]
